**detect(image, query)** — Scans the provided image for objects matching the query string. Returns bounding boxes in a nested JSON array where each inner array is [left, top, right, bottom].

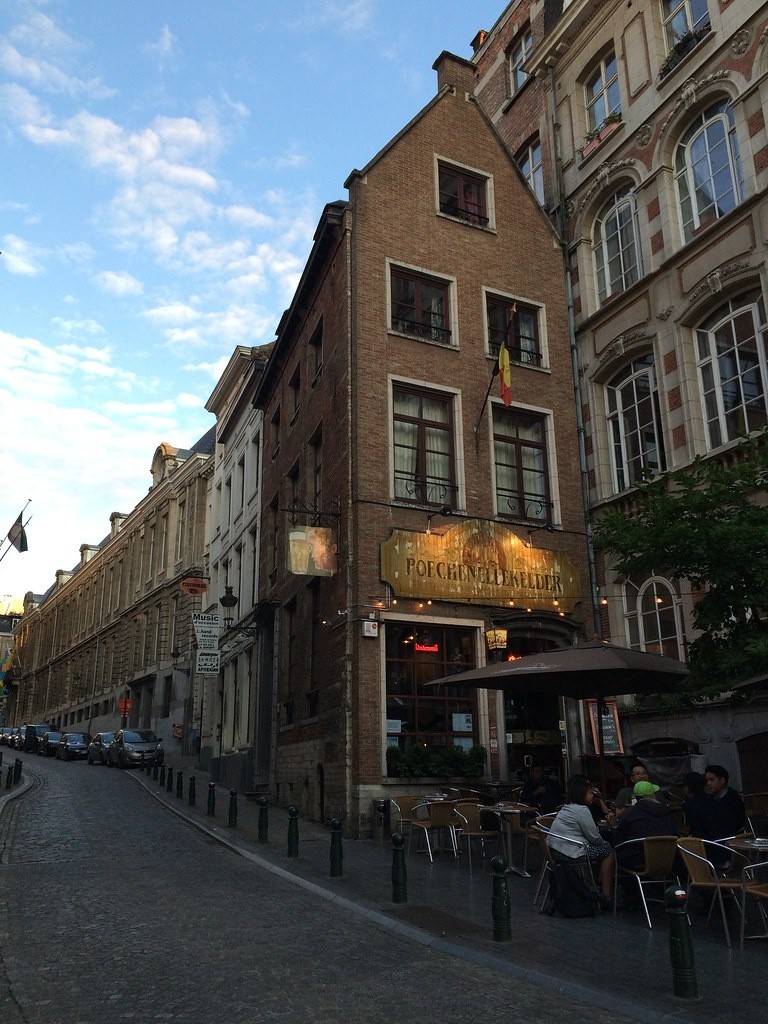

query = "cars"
[[55, 733, 95, 760], [0, 727, 27, 751], [108, 728, 164, 769], [36, 731, 70, 756], [87, 731, 117, 767]]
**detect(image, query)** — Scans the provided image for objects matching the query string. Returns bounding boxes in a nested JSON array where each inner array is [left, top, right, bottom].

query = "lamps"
[[528, 525, 554, 536], [427, 506, 451, 525], [485, 625, 509, 659], [220, 585, 258, 637], [171, 649, 189, 674], [71, 674, 87, 691], [27, 683, 37, 697]]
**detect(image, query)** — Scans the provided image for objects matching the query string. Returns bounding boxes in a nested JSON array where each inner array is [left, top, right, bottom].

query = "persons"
[[547, 774, 617, 913], [615, 765, 666, 807], [521, 765, 565, 814], [112, 731, 124, 746], [676, 768, 745, 916], [615, 780, 676, 917]]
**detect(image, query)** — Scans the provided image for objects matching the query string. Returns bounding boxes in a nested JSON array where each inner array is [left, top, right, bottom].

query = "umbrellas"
[[422, 635, 705, 756]]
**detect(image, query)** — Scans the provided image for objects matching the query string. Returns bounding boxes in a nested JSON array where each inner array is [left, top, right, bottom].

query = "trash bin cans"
[[371, 797, 391, 840]]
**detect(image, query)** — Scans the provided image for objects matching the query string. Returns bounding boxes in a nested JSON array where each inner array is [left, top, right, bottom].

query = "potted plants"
[[660, 26, 706, 77], [577, 114, 622, 159]]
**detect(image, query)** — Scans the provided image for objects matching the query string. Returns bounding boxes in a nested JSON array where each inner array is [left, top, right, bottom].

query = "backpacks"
[[548, 860, 608, 918]]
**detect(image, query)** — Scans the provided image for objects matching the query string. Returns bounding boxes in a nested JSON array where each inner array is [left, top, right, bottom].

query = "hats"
[[634, 781, 659, 796]]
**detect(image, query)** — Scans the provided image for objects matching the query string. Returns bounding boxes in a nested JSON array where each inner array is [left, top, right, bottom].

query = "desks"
[[726, 837, 768, 934], [598, 816, 640, 906], [411, 792, 460, 857], [481, 805, 536, 876]]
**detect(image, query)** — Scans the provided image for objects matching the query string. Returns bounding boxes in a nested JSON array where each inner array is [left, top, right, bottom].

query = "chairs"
[[388, 790, 768, 953]]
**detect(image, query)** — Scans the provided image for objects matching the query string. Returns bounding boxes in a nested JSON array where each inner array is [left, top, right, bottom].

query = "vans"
[[24, 724, 58, 753]]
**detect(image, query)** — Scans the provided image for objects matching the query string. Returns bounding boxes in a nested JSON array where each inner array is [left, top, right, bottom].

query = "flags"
[[498, 323, 512, 407], [7, 511, 28, 552]]
